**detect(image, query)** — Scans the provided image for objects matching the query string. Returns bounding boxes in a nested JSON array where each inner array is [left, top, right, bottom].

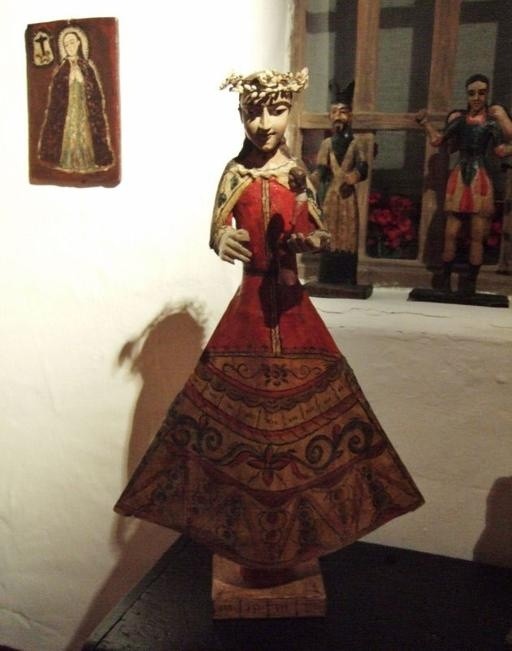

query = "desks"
[[84, 535, 512, 650]]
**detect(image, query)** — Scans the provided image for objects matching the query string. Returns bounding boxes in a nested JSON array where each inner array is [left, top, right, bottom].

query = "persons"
[[109, 68, 429, 589], [308, 77, 369, 288], [36, 26, 118, 176], [414, 72, 511, 300]]
